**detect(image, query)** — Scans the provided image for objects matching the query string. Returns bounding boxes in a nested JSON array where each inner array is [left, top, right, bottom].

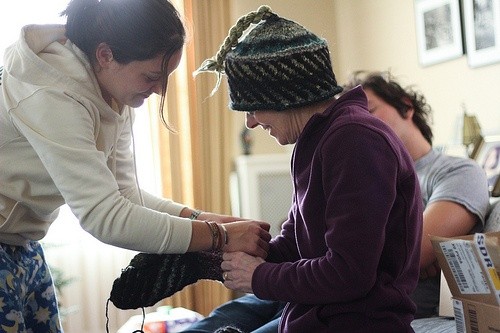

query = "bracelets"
[[204, 220, 222, 252], [189, 209, 202, 219], [219, 222, 228, 250]]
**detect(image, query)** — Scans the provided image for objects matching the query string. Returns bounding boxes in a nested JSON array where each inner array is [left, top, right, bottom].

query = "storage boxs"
[[427, 231, 500, 333]]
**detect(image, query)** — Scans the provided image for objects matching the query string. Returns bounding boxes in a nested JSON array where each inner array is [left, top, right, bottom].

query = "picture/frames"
[[470, 135, 500, 197], [460, 0, 499, 67], [415, 0, 463, 67]]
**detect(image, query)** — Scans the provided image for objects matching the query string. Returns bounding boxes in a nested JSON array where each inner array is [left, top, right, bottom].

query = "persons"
[[0, 0, 272, 333], [180, 69, 490, 333], [194, 5, 423, 333]]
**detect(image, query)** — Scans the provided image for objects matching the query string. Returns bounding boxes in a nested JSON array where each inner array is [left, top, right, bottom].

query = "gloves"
[[109, 245, 230, 309]]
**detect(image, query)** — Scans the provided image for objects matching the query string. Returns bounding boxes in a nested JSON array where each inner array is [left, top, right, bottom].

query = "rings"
[[223, 271, 228, 280]]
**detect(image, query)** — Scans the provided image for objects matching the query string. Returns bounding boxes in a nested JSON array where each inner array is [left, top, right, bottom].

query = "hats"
[[194, 5, 343, 111]]
